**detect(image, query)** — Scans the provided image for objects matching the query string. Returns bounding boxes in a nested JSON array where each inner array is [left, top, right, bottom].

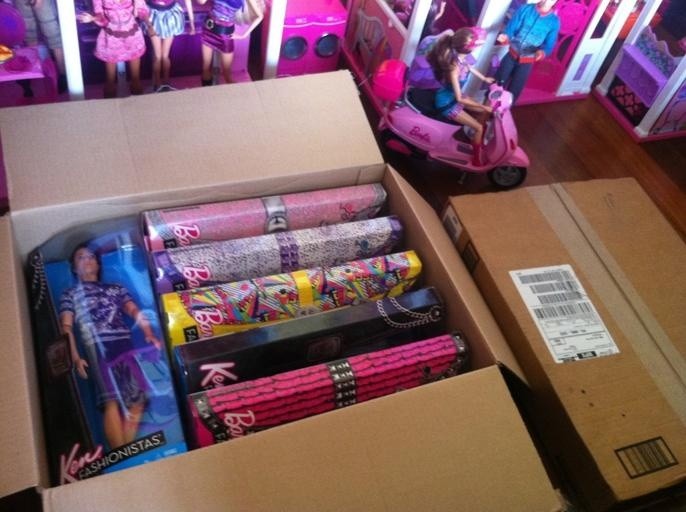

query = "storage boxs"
[[1, 69, 565, 512], [439, 177, 686, 512]]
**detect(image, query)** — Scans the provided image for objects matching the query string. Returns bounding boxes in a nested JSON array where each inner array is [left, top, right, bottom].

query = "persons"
[[434, 26, 500, 167], [58, 246, 162, 452], [74, 1, 155, 96], [494, 0, 561, 108], [183, 1, 268, 90], [143, 0, 196, 90]]
